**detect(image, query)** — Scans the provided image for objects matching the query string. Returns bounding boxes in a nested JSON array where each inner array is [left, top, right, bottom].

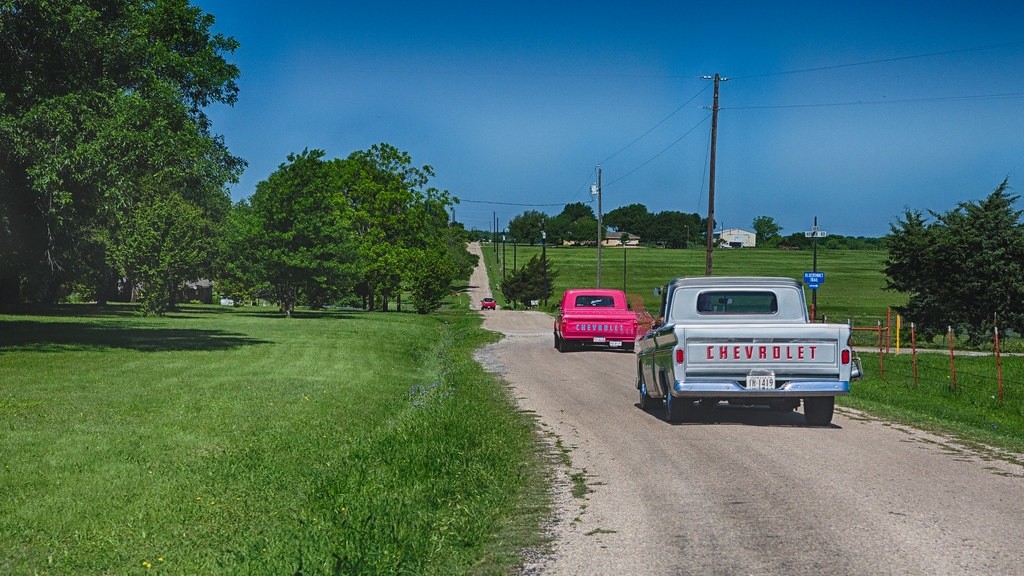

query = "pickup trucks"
[[636, 275, 853, 428], [554, 288, 638, 351]]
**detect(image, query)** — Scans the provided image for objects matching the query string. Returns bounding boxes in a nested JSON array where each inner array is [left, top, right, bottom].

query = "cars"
[[480, 298, 496, 311]]
